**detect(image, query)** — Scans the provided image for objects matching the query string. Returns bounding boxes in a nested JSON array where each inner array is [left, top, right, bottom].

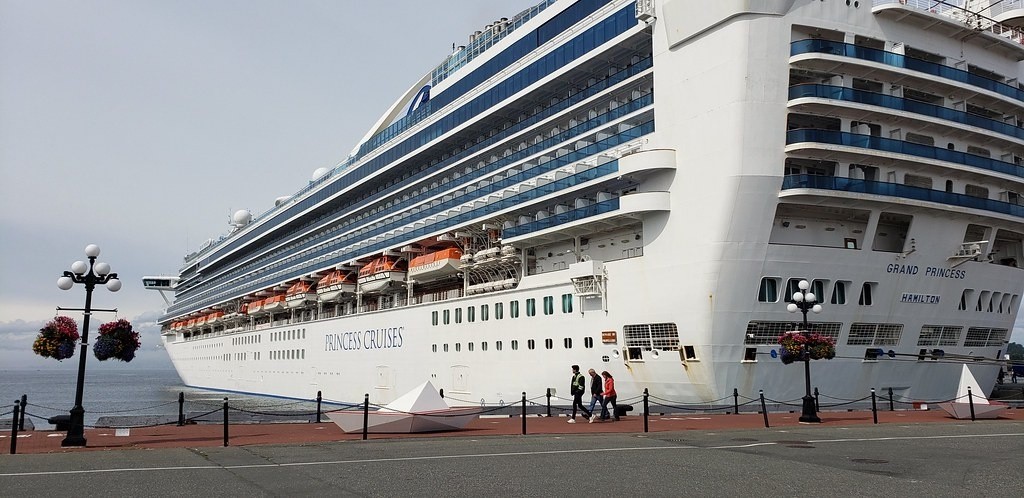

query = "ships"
[[142, 1, 1024, 418]]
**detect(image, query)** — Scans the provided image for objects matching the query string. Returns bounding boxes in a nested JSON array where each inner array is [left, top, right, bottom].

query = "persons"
[[600, 371, 620, 421], [582, 369, 610, 420], [1011, 368, 1017, 384], [567, 365, 596, 424]]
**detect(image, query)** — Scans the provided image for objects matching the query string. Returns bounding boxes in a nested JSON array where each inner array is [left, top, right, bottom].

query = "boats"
[[316, 269, 357, 304], [176, 320, 189, 334], [169, 322, 178, 332], [196, 316, 210, 330], [407, 247, 463, 287], [323, 380, 483, 435], [284, 279, 317, 311], [264, 294, 288, 314], [187, 318, 198, 332], [247, 298, 268, 319], [357, 255, 408, 297], [208, 311, 225, 327]]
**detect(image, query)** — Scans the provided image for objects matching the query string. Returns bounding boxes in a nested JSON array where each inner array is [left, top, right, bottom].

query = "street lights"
[[56, 245, 123, 447], [786, 280, 824, 424]]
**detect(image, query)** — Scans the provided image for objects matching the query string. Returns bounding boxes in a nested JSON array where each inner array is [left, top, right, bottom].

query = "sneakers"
[[567, 419, 576, 424], [589, 414, 596, 423]]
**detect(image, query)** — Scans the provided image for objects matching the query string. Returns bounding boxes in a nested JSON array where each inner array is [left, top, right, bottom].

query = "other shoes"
[[597, 417, 605, 421], [613, 418, 620, 421], [605, 415, 610, 419], [582, 413, 589, 420]]
